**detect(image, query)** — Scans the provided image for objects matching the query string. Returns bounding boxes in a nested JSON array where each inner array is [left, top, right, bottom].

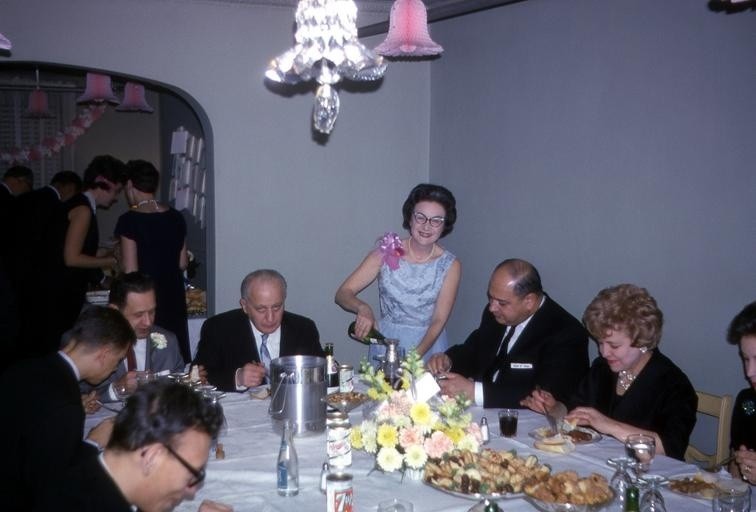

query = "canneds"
[[326, 421, 351, 468], [339, 364, 354, 393], [326, 411, 348, 425], [327, 474, 352, 511]]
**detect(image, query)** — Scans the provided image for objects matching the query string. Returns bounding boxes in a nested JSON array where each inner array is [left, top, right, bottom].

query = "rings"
[[745, 465, 751, 473], [743, 475, 748, 481]]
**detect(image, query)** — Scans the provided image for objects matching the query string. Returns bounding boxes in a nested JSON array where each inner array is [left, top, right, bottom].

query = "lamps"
[[114, 80, 154, 112], [20, 68, 56, 120], [264, 0, 388, 136], [372, 0, 445, 57], [76, 72, 121, 105]]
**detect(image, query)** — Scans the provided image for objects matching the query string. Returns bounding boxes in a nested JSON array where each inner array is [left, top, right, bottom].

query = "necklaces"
[[617, 369, 638, 391], [409, 236, 435, 263]]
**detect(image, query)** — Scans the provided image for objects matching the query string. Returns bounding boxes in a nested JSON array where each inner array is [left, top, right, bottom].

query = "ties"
[[125, 343, 137, 372], [260, 334, 272, 384], [489, 325, 515, 383]]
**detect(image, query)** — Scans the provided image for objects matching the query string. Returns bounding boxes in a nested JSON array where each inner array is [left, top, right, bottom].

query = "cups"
[[137, 373, 159, 386], [499, 410, 519, 438], [712, 478, 751, 511], [378, 501, 414, 512]]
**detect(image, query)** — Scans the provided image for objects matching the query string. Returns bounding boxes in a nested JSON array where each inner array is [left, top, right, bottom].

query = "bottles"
[[624, 485, 641, 510], [480, 416, 492, 442], [348, 321, 388, 345], [324, 341, 340, 390], [383, 340, 403, 391], [320, 463, 330, 491], [276, 419, 299, 497]]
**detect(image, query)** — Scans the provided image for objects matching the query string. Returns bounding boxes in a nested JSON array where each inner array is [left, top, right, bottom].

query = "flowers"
[[149, 332, 168, 373], [349, 342, 484, 484]]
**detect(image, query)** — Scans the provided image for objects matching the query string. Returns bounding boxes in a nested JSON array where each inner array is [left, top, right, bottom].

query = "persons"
[[192, 269, 326, 394], [520, 284, 698, 462], [427, 258, 590, 420], [0, 155, 192, 402], [334, 185, 460, 376], [726, 302, 756, 487], [2, 305, 137, 474], [2, 378, 229, 512]]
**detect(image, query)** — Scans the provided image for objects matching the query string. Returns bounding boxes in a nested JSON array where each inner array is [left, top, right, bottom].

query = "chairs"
[[684, 391, 734, 472]]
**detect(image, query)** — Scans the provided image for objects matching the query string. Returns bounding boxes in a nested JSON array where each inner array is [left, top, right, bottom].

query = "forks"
[[700, 450, 753, 474], [536, 385, 557, 435]]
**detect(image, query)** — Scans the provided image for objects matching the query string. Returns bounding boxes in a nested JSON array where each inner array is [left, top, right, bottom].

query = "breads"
[[562, 414, 577, 434], [534, 435, 573, 455]]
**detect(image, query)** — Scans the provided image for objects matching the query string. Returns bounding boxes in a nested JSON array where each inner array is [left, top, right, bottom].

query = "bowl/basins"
[[524, 480, 612, 511], [85, 292, 112, 305]]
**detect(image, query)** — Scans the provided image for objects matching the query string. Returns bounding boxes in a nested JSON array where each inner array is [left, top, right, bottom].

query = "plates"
[[529, 425, 602, 445], [660, 470, 752, 502], [427, 483, 523, 501], [98, 239, 119, 249]]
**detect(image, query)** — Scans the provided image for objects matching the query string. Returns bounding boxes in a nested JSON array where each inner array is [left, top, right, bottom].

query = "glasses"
[[163, 443, 206, 488], [412, 211, 445, 228]]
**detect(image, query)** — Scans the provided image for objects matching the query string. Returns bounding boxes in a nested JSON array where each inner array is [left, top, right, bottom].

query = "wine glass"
[[606, 455, 634, 510], [180, 380, 201, 389], [204, 391, 229, 438], [637, 471, 669, 511], [625, 436, 655, 488], [195, 385, 218, 399], [168, 372, 189, 383], [112, 384, 133, 408]]
